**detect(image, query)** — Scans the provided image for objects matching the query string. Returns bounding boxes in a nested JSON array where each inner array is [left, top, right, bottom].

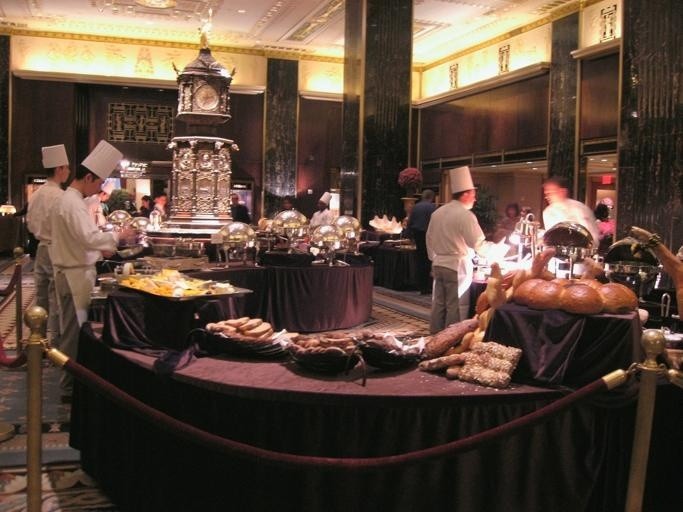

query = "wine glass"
[[216, 212, 360, 267]]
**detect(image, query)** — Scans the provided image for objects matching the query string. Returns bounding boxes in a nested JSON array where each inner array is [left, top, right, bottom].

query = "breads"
[[205, 246, 640, 385]]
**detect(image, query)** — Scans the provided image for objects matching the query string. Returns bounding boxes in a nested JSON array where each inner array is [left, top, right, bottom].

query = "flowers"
[[398, 166, 424, 197]]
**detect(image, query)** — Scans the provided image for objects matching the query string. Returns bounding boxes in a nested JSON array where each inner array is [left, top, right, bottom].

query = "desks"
[[65, 318, 683, 511], [359, 239, 421, 291], [114, 257, 375, 333]]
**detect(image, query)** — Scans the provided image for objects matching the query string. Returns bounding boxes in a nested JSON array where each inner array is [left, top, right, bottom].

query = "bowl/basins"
[[191, 249, 204, 257], [151, 243, 176, 257], [368, 241, 380, 247]]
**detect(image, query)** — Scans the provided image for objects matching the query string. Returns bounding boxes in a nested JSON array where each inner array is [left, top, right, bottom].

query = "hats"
[[449, 165, 479, 194], [40, 143, 69, 169], [320, 191, 332, 206], [80, 139, 125, 182]]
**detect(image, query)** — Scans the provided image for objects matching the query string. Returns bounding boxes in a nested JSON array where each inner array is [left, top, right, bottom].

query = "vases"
[[402, 198, 418, 223]]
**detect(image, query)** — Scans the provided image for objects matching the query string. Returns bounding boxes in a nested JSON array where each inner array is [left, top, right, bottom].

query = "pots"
[[115, 244, 142, 259]]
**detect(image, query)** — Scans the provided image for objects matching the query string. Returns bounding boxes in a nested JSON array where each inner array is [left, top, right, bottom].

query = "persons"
[[541, 175, 599, 245], [593, 203, 616, 240], [26, 144, 70, 341], [149, 195, 167, 220], [424, 164, 507, 336], [52, 141, 132, 404], [139, 195, 153, 212], [152, 197, 159, 204], [281, 196, 293, 211], [84, 179, 115, 225], [405, 188, 438, 295], [513, 206, 533, 255], [308, 191, 333, 224], [230, 193, 250, 224], [629, 225, 683, 322], [491, 203, 524, 253]]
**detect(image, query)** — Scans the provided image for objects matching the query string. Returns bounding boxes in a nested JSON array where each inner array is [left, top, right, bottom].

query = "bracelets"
[[641, 234, 663, 249]]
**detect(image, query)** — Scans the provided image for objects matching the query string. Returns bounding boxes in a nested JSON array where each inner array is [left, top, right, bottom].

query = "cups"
[[98, 277, 114, 298]]
